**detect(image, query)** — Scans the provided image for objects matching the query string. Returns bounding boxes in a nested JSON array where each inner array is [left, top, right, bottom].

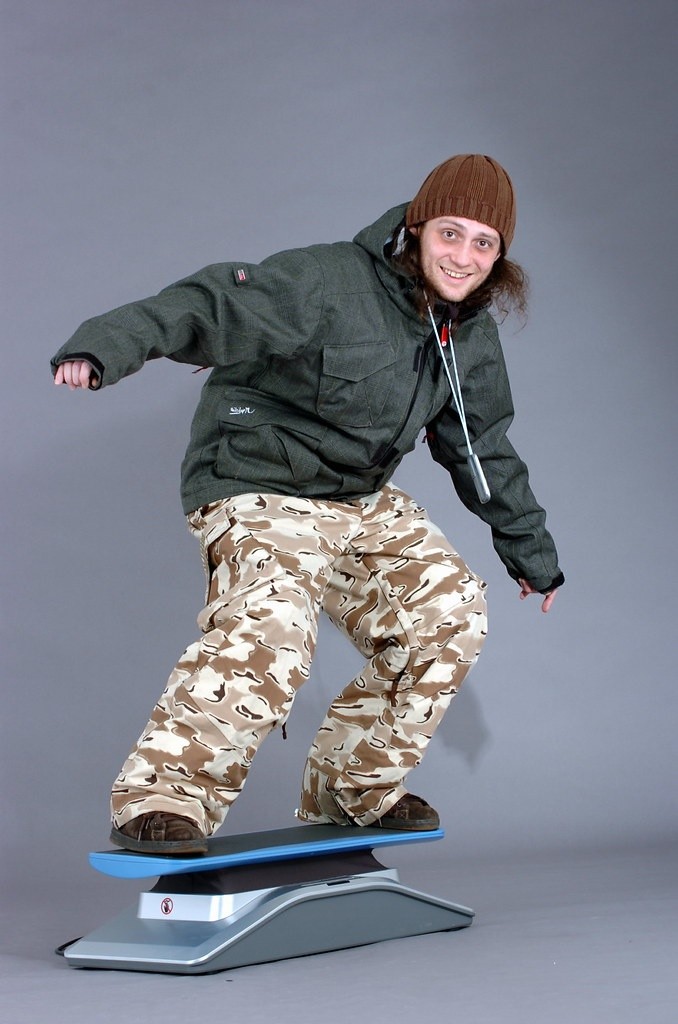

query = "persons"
[[46, 151, 570, 859]]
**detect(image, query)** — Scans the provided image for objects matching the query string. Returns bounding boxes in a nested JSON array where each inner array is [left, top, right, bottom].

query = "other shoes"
[[370, 793, 439, 830], [108, 809, 209, 853]]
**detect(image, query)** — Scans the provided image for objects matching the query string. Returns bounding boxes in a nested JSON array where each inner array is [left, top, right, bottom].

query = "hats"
[[407, 155, 517, 261]]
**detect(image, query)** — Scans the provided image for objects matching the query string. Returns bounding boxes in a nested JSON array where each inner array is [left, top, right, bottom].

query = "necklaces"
[[417, 289, 493, 512]]
